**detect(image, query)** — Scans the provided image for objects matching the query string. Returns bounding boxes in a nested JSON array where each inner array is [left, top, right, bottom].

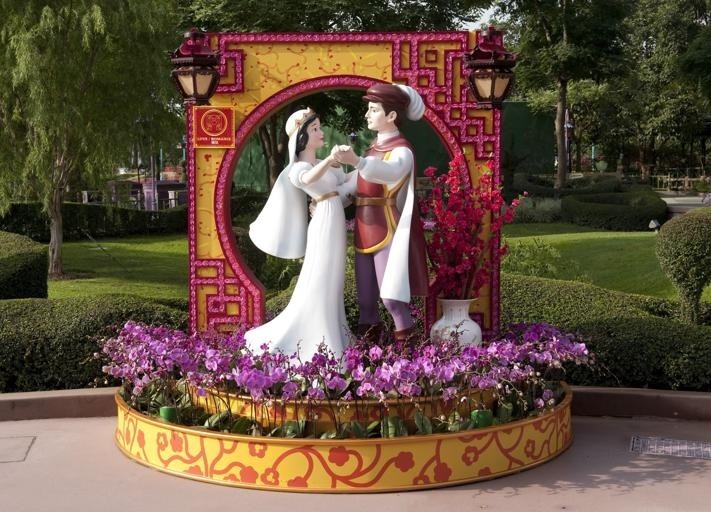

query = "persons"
[[239, 107, 355, 371], [307, 81, 431, 348]]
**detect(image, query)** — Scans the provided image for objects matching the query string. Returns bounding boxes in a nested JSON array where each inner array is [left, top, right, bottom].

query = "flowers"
[[94, 319, 593, 439], [413, 149, 529, 296]]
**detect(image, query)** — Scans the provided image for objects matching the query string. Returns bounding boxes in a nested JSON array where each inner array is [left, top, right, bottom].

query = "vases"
[[430, 297, 482, 344]]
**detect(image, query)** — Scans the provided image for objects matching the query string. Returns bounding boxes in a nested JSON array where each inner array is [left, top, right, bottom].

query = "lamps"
[[169, 27, 222, 106], [463, 25, 517, 109]]
